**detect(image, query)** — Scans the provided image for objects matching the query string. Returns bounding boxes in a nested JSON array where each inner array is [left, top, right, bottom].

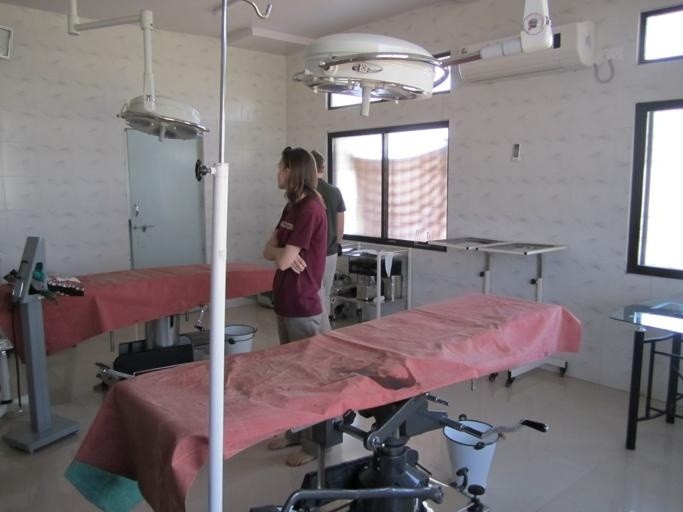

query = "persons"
[[311, 150, 346, 317], [263, 147, 343, 466]]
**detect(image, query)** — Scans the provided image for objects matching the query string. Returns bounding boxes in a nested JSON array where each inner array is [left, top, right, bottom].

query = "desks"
[[604, 296, 683, 450]]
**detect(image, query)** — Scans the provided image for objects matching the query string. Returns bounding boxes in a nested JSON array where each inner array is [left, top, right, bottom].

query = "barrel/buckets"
[[224, 323, 258, 355], [441, 413, 501, 498]]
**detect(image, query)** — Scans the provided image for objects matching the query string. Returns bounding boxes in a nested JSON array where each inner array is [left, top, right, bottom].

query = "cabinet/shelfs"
[[329, 243, 413, 318]]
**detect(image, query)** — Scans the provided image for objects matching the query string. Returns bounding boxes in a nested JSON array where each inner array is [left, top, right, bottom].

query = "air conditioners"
[[455, 21, 598, 83]]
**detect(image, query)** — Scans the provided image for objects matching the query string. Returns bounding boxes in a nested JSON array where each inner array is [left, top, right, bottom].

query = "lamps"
[[68, 8, 211, 145], [292, 0, 557, 117]]
